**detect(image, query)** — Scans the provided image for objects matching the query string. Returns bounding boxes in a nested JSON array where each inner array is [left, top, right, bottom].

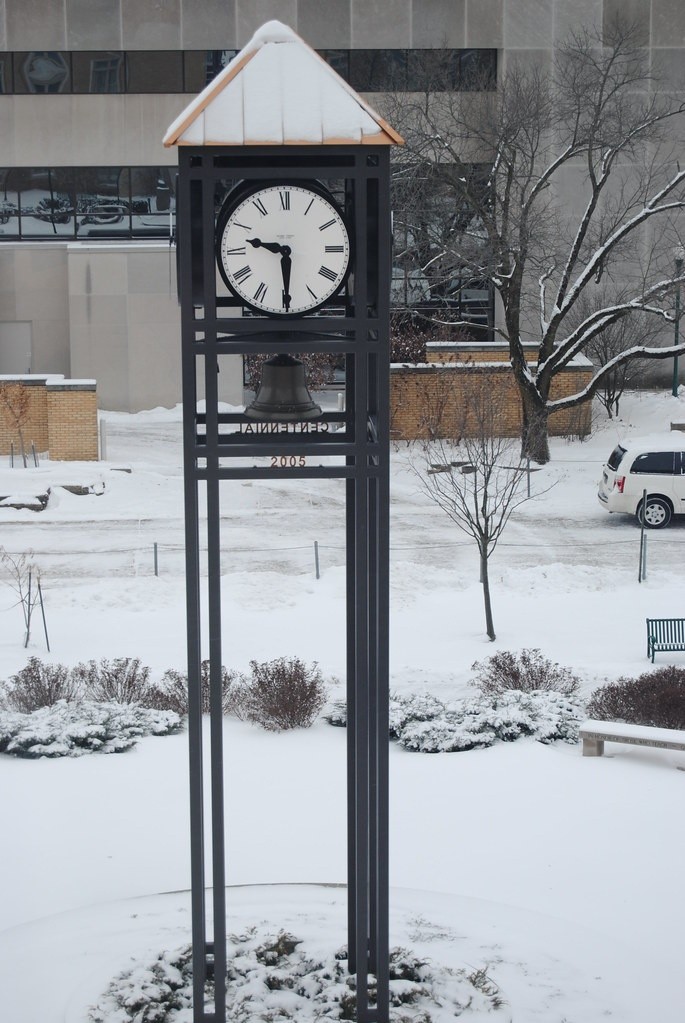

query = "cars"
[[597, 432, 685, 529]]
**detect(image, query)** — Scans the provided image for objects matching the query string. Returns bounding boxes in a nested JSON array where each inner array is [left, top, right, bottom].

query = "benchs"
[[646, 618, 685, 663]]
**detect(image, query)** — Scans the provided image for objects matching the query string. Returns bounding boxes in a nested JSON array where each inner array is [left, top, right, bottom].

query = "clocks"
[[215, 180, 354, 320]]
